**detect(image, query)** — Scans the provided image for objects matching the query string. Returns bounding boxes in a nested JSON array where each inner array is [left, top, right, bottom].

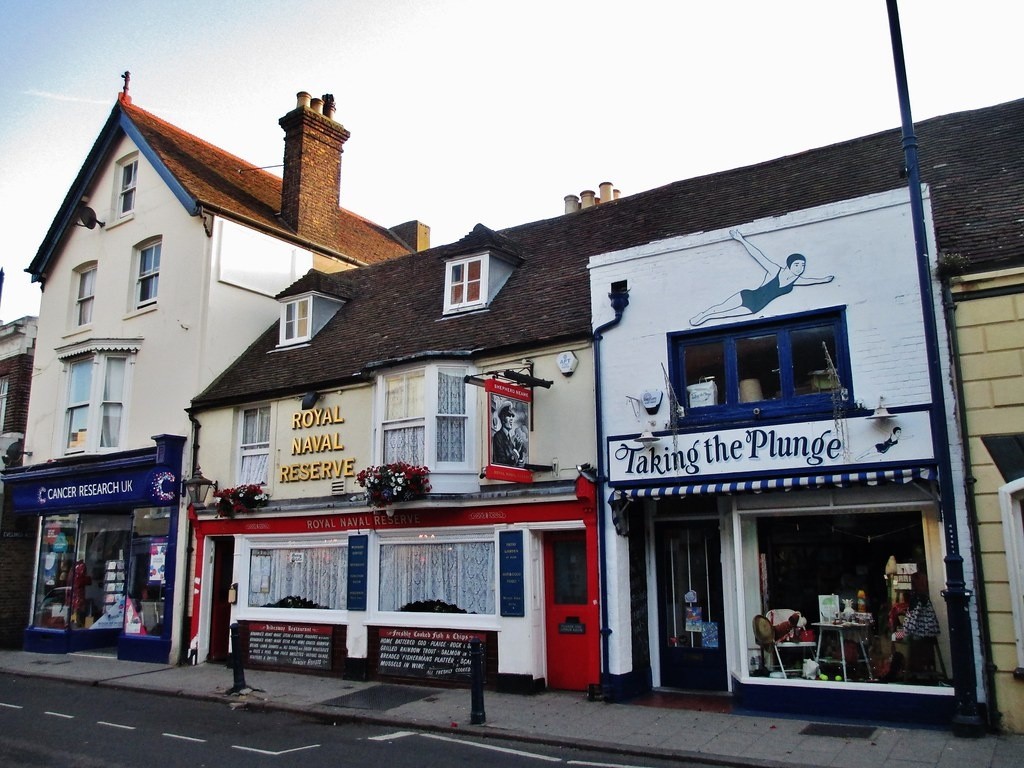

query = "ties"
[[508, 434, 515, 451]]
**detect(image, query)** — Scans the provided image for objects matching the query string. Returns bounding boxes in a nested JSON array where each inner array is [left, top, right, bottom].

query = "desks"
[[810, 621, 873, 682]]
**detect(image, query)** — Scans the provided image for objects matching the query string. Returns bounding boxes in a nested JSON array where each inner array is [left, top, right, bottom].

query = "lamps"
[[1, 451, 32, 466], [183, 463, 219, 510], [634, 421, 661, 448], [302, 391, 322, 410], [865, 397, 898, 419], [576, 463, 591, 473], [580, 468, 597, 482]]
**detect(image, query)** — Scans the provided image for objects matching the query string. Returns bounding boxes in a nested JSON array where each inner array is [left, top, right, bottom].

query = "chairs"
[[765, 609, 822, 679]]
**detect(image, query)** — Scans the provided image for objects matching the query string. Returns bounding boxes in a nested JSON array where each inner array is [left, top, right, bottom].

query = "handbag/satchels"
[[43, 522, 69, 553], [902, 594, 941, 637]]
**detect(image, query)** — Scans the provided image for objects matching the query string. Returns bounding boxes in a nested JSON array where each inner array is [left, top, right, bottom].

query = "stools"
[[891, 636, 947, 683]]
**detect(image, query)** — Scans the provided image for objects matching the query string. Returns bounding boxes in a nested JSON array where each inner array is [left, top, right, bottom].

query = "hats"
[[498, 401, 515, 418], [45, 552, 56, 570]]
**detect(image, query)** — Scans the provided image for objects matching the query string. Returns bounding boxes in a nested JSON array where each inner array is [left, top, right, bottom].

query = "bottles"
[[857, 590, 868, 624]]
